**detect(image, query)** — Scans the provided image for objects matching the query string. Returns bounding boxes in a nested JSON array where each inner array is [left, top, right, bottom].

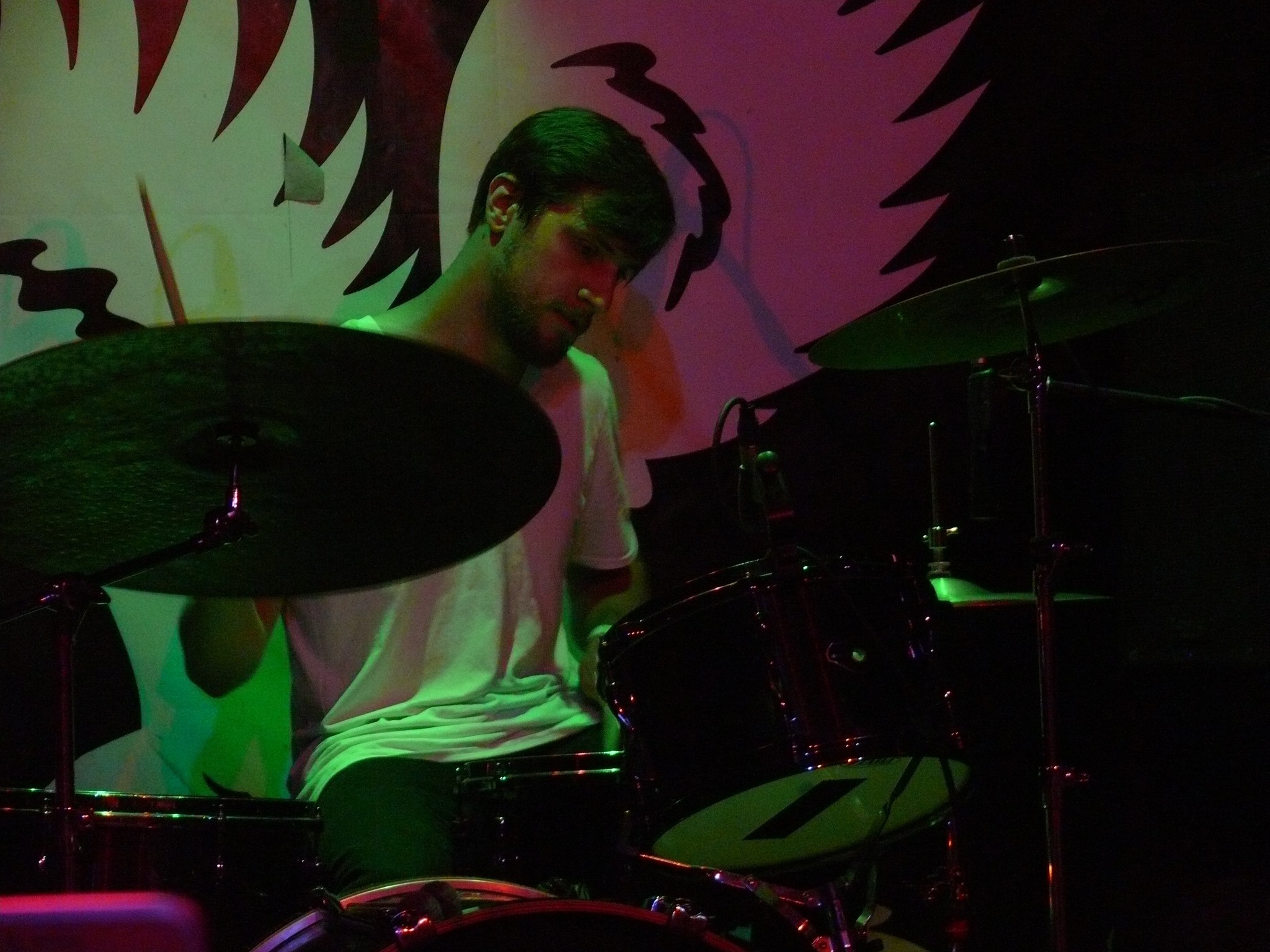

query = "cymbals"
[[808, 240, 1251, 371], [929, 578, 1114, 605], [0, 322, 561, 599]]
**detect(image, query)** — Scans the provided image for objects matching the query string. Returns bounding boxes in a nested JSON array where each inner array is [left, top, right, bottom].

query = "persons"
[[176, 106, 677, 901]]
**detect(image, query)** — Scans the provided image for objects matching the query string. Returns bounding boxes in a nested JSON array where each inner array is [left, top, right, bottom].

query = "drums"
[[247, 876, 745, 952], [0, 788, 124, 894], [97, 794, 323, 952], [596, 546, 974, 879], [450, 750, 708, 914]]
